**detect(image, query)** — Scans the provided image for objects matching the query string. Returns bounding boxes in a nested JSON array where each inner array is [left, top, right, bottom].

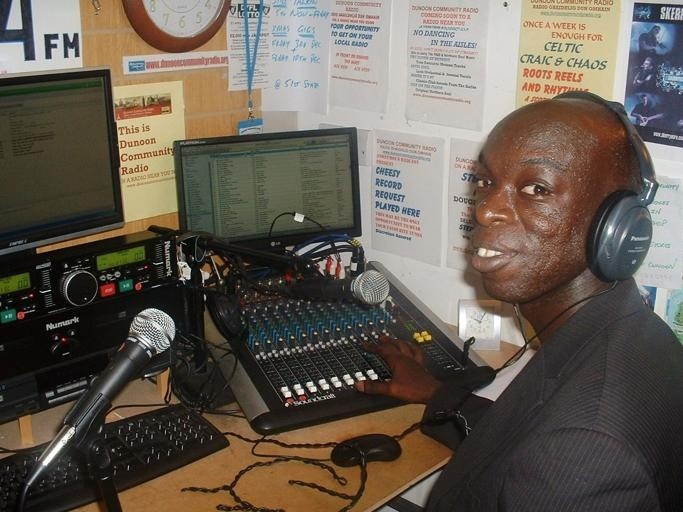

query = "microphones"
[[25, 306, 176, 496], [282, 270, 391, 304]]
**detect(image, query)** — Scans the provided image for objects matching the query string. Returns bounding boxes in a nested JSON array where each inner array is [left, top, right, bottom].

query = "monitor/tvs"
[[173, 127, 363, 253], [0, 67, 125, 256]]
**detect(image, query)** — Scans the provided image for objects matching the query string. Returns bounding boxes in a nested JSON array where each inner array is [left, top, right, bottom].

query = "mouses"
[[333, 432, 402, 467]]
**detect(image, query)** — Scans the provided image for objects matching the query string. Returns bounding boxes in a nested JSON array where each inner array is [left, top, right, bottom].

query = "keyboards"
[[1, 402, 231, 512]]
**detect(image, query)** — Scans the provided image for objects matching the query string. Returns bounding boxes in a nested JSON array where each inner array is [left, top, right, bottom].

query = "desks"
[[0, 322, 539, 511]]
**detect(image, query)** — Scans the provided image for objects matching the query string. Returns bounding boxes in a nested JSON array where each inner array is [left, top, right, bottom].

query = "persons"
[[632, 57, 657, 93], [631, 95, 665, 128], [637, 25, 666, 63], [353, 95, 682, 512]]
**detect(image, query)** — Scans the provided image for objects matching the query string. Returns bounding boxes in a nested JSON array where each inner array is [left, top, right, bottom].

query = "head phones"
[[173, 230, 245, 341], [552, 89, 661, 285]]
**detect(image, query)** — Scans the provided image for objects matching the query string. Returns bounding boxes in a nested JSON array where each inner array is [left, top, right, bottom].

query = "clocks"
[[462, 304, 496, 344], [120, 1, 231, 54]]
